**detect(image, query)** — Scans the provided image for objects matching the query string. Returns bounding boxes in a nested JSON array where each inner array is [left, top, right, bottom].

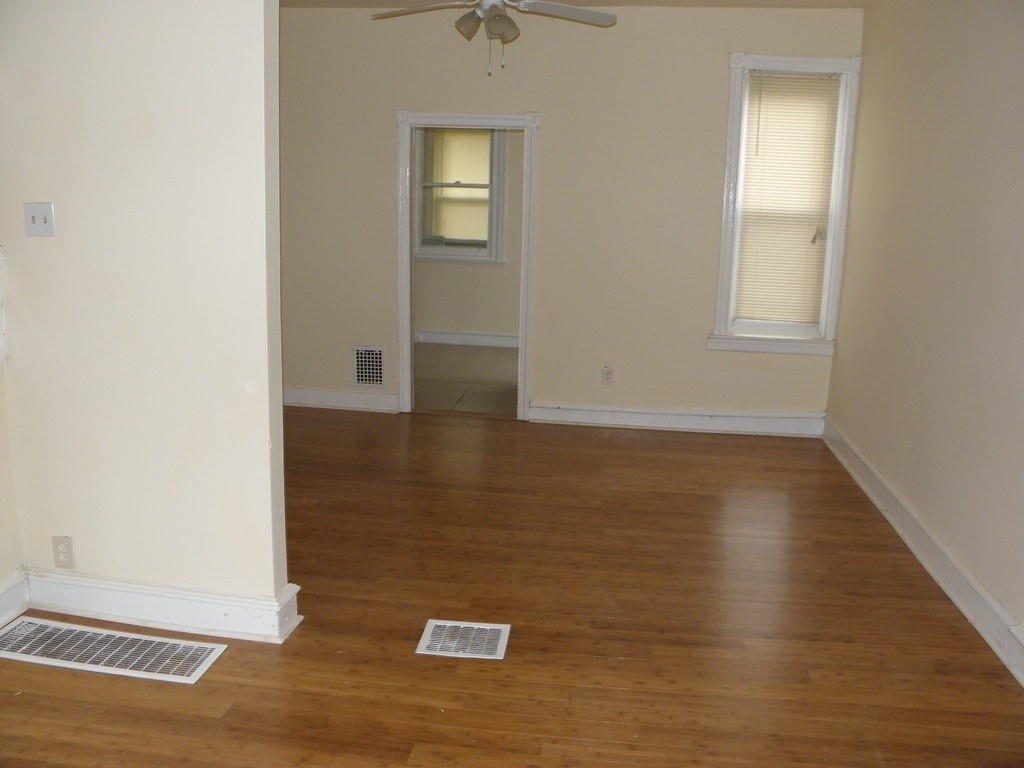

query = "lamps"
[[455, 4, 520, 45]]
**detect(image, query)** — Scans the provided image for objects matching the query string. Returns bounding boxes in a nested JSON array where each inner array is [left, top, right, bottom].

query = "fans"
[[371, 0, 617, 28]]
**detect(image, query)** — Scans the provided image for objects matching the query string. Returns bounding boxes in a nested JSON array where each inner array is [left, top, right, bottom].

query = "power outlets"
[[52, 535, 75, 569]]
